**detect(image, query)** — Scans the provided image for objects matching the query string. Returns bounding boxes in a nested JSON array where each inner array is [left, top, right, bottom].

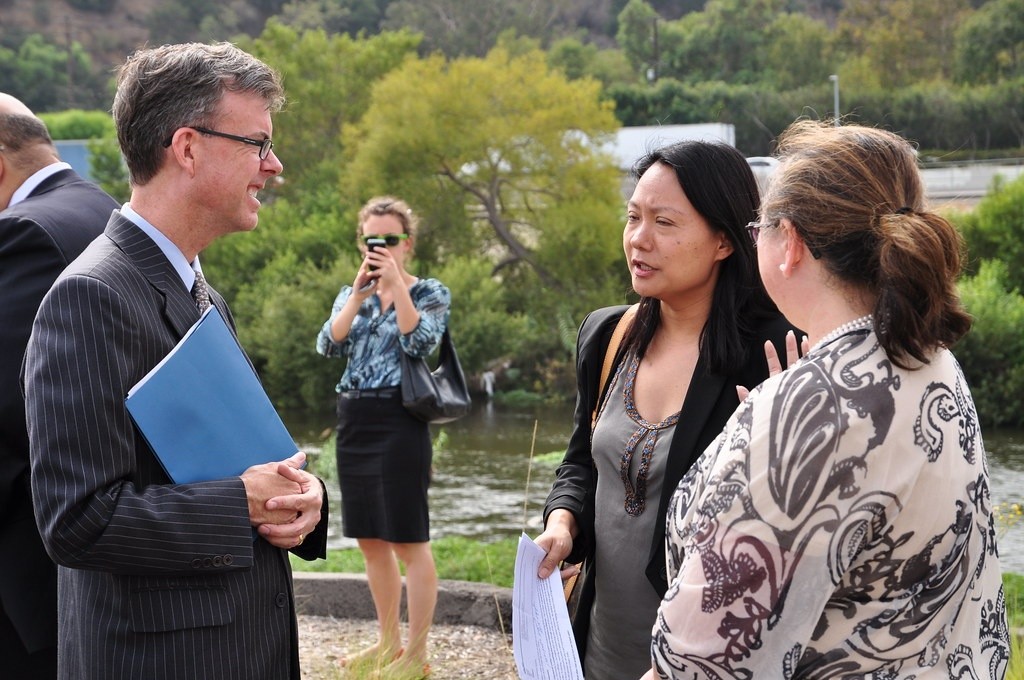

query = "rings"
[[298, 534, 303, 546]]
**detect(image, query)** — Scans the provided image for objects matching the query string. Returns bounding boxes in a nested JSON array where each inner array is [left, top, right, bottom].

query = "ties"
[[193, 270, 212, 314]]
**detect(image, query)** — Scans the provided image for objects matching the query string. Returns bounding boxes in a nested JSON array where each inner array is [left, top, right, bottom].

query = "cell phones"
[[366, 238, 385, 280]]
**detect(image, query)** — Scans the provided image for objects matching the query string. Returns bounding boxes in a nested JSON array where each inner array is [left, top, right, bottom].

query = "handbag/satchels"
[[400, 280, 471, 424], [557, 555, 589, 626]]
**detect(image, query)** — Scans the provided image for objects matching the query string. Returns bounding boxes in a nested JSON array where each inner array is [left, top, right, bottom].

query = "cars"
[[745, 156, 780, 174]]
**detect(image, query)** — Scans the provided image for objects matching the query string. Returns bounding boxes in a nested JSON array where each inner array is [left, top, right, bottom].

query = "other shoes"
[[367, 660, 429, 680], [333, 647, 404, 670]]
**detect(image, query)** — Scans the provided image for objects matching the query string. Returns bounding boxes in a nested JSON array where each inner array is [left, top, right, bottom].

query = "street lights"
[[830, 75, 839, 129]]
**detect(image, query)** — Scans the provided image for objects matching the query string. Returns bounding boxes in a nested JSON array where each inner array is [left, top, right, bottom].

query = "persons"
[[314, 194, 452, 679], [530, 141, 808, 680], [0, 90, 121, 680], [650, 123, 1011, 680], [18, 42, 328, 680]]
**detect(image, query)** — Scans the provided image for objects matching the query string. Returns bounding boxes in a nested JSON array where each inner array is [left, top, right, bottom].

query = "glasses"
[[745, 216, 822, 259], [361, 234, 408, 246], [162, 126, 274, 161]]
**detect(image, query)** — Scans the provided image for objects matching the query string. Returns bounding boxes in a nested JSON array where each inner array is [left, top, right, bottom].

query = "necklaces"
[[807, 312, 891, 353]]
